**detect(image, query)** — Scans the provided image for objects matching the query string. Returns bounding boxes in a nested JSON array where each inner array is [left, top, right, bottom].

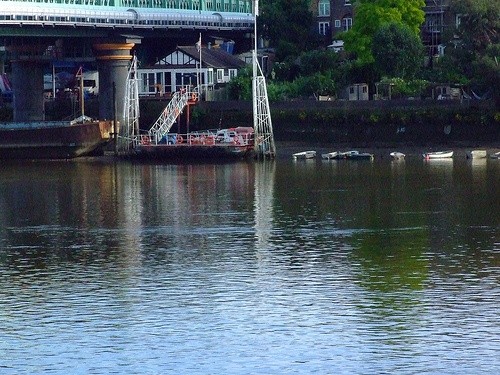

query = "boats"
[[423, 149, 453, 159], [143, 121, 256, 158], [321, 149, 347, 160], [293, 149, 317, 160], [388, 150, 404, 159], [344, 152, 374, 159], [0, 66, 125, 156], [465, 148, 487, 159]]
